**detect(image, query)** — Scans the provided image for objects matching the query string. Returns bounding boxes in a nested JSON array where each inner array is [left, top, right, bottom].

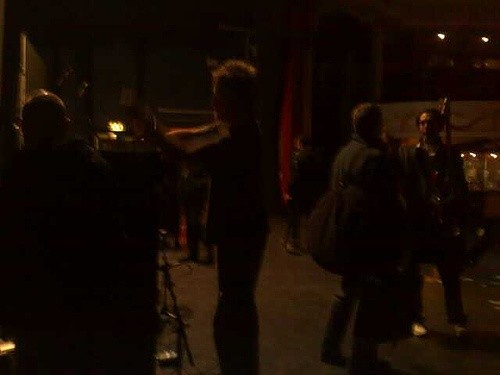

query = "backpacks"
[[308, 147, 392, 275]]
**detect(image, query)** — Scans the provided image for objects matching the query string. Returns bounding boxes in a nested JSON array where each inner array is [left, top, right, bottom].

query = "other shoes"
[[450, 321, 469, 337], [280, 238, 306, 256], [179, 253, 213, 265], [409, 321, 428, 336], [320, 355, 351, 368], [352, 360, 392, 374]]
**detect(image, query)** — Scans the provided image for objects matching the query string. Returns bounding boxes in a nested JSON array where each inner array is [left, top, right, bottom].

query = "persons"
[[1, 94, 157, 375], [284, 136, 325, 256], [126, 104, 216, 266], [304, 105, 404, 375], [400, 109, 487, 339], [134, 61, 270, 375], [0, 103, 24, 165]]
